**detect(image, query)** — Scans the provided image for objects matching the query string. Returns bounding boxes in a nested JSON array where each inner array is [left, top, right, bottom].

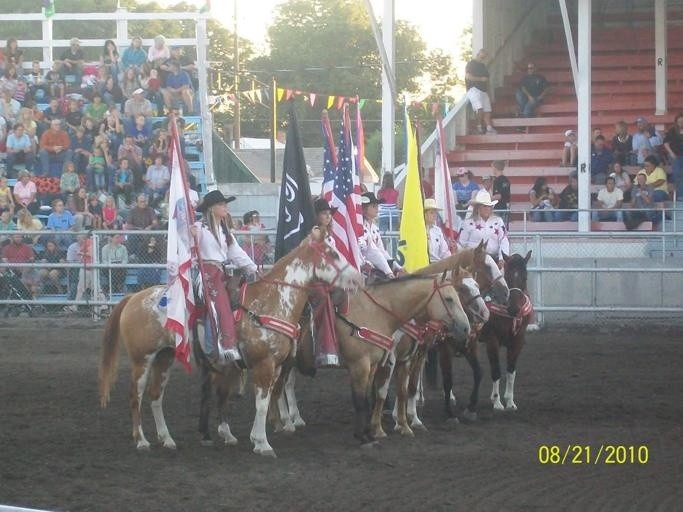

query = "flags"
[[273, 95, 318, 264], [166, 111, 218, 369], [322, 94, 366, 272], [412, 116, 424, 197], [397, 92, 429, 276], [434, 117, 461, 240]]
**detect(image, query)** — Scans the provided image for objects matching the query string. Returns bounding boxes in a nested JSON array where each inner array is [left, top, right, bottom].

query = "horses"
[[196, 267, 474, 450], [476, 247, 534, 414], [405, 238, 511, 428], [223, 260, 493, 427], [96, 216, 366, 465]]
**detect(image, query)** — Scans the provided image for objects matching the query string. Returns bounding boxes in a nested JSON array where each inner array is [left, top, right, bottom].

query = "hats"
[[455, 166, 468, 176], [132, 87, 145, 97], [312, 198, 336, 212], [469, 190, 498, 207], [633, 117, 648, 126], [358, 191, 383, 204], [192, 188, 237, 212], [422, 196, 445, 213]]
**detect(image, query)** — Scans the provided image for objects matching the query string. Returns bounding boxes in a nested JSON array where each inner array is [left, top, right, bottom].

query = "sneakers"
[[61, 304, 77, 313]]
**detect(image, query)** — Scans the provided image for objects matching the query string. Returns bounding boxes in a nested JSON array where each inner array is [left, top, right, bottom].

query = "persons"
[[513, 60, 547, 132], [0, 30, 202, 315], [233, 211, 273, 265], [529, 107, 681, 229], [353, 192, 404, 281], [451, 166, 479, 207], [458, 186, 509, 270], [376, 171, 399, 203], [486, 159, 512, 221], [464, 49, 498, 135], [423, 197, 452, 268], [304, 198, 343, 370], [189, 189, 260, 373]]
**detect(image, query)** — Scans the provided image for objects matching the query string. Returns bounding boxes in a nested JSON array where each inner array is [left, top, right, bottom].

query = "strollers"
[[0, 267, 43, 318]]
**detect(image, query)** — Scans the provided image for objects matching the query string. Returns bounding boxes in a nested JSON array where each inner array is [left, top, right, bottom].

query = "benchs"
[[35, 104, 207, 287]]
